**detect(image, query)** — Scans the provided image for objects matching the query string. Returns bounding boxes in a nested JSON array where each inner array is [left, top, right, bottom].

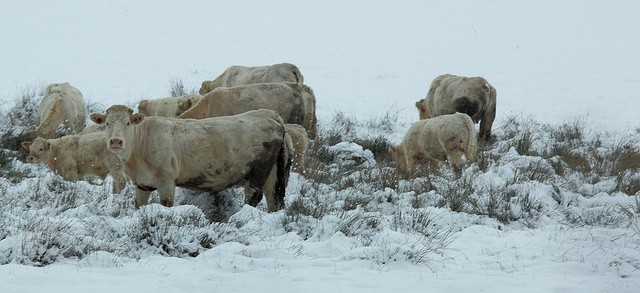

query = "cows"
[[283, 123, 308, 174], [389, 112, 477, 180], [199, 63, 304, 96], [34, 82, 86, 139], [89, 105, 291, 213], [415, 74, 497, 142], [21, 132, 127, 194], [137, 95, 203, 119], [176, 81, 317, 141]]
